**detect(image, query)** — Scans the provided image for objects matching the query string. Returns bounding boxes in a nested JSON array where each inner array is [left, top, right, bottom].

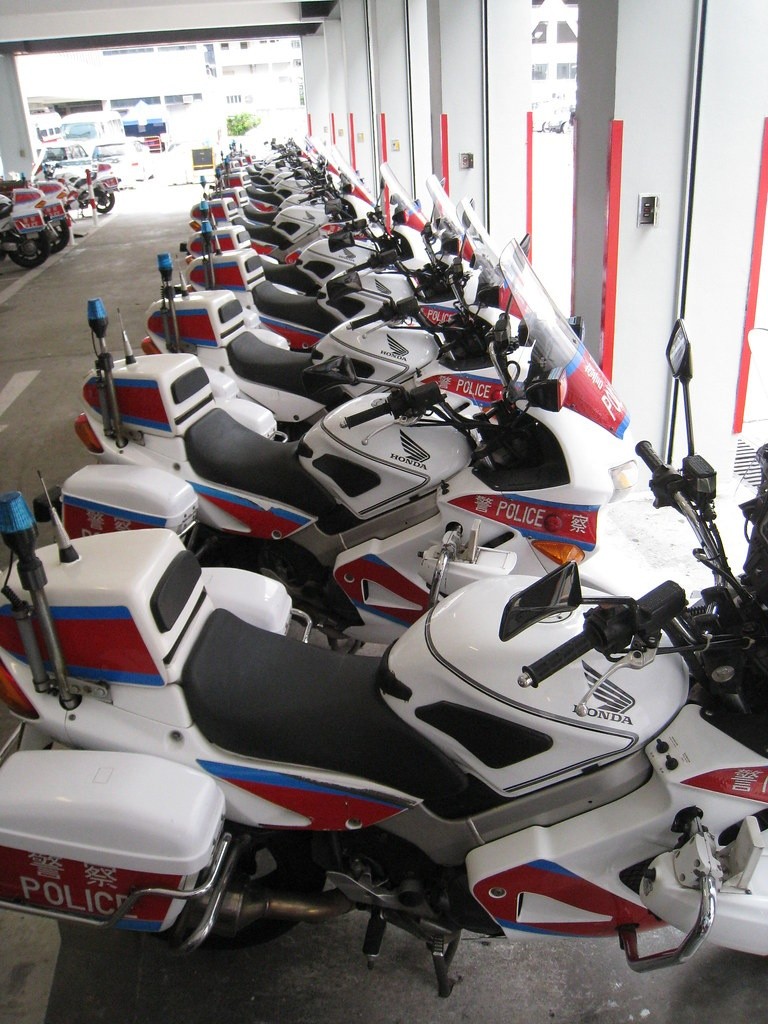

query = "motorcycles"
[[0, 102, 768, 1004]]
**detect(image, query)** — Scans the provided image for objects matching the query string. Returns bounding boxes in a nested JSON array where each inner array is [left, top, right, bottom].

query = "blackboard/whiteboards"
[[191, 147, 215, 171]]
